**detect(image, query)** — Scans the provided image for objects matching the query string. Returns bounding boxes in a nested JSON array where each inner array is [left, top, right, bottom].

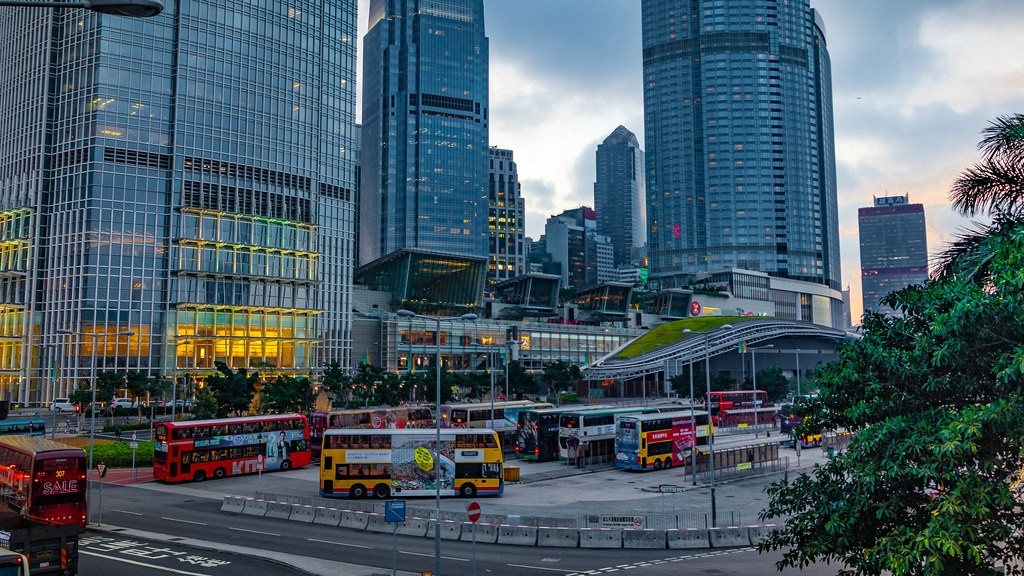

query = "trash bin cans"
[[503, 466, 520, 481]]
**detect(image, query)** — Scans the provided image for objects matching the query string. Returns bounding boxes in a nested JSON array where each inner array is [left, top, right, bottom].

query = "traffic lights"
[[76, 406, 80, 413]]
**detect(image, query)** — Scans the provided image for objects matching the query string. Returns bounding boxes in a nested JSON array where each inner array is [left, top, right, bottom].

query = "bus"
[[311, 406, 433, 459], [0, 419, 46, 436], [319, 429, 505, 500], [152, 414, 311, 483], [0, 435, 87, 534], [441, 389, 869, 472], [0, 502, 79, 576]]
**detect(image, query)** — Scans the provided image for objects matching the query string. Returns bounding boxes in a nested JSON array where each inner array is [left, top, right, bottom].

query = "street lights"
[[469, 341, 515, 428], [732, 345, 775, 438], [56, 330, 136, 525], [682, 324, 733, 488], [796, 348, 800, 396], [395, 308, 478, 576]]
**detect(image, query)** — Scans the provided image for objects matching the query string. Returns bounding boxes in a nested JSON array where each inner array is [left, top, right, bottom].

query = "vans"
[[50, 398, 76, 413]]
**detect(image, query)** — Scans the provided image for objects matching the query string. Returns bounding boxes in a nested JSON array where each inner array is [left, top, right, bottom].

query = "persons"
[[772, 414, 776, 426], [115, 426, 121, 441], [576, 442, 585, 469], [176, 411, 181, 419], [277, 431, 290, 460], [146, 409, 155, 419], [127, 413, 129, 422], [187, 411, 192, 418]]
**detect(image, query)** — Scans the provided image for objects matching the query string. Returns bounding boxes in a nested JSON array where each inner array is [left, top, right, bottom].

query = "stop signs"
[[468, 502, 481, 522]]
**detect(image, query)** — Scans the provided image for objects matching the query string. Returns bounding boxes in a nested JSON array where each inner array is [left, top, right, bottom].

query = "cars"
[[159, 399, 198, 407], [111, 398, 146, 409]]
